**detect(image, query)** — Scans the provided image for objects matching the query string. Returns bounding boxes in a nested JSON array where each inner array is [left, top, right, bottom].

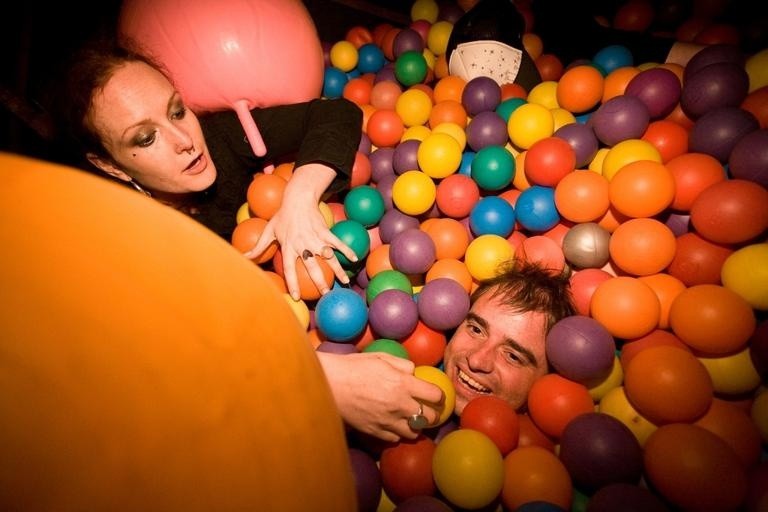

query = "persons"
[[54, 39, 446, 444], [444, 260, 575, 419]]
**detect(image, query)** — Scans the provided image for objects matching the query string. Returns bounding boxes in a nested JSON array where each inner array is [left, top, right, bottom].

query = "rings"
[[317, 246, 336, 260], [409, 401, 430, 433]]
